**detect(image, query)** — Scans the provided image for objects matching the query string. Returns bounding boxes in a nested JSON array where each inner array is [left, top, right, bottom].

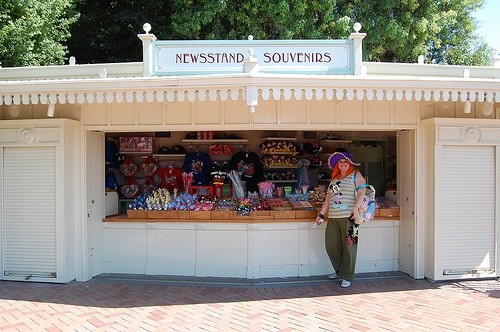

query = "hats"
[[328, 151, 361, 170]]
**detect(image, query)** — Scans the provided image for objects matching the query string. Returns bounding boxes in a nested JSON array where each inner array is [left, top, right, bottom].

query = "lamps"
[[464, 100, 471, 114], [47, 105, 56, 117]]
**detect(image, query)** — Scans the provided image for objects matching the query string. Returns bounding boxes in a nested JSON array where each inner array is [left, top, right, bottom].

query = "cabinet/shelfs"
[[262, 138, 351, 183], [153, 139, 249, 156]]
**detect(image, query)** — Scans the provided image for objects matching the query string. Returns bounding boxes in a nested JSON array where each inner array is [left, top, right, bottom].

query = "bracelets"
[[318, 214, 324, 219]]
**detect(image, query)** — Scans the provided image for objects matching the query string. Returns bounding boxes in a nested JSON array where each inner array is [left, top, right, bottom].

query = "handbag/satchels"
[[353, 170, 376, 223]]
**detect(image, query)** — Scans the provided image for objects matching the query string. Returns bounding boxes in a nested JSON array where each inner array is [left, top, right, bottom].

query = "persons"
[[315, 151, 366, 287]]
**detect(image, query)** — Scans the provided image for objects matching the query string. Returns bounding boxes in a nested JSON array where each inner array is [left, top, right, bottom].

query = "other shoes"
[[341, 280, 351, 287], [328, 271, 340, 278]]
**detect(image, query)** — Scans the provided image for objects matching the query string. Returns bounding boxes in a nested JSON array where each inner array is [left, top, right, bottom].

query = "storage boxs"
[[375, 209, 399, 216], [127, 201, 328, 220]]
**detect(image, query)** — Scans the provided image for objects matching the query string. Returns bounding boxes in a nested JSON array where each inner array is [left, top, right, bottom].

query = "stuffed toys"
[[363, 201, 376, 221]]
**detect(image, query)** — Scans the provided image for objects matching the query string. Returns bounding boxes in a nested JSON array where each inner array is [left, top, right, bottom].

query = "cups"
[[284, 187, 292, 195], [277, 187, 283, 197]]
